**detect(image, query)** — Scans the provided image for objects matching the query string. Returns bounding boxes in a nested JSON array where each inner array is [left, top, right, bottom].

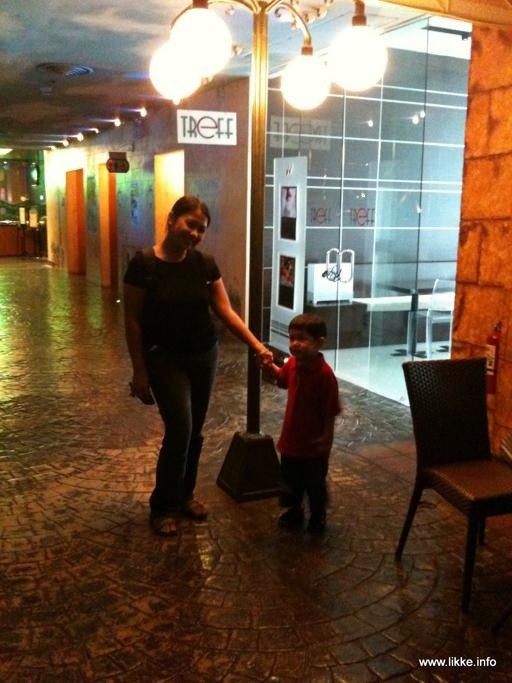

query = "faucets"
[[149, 510, 177, 537], [185, 500, 208, 518]]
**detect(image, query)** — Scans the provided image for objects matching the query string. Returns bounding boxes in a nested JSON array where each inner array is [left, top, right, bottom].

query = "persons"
[[119, 195, 277, 535], [254, 312, 342, 542]]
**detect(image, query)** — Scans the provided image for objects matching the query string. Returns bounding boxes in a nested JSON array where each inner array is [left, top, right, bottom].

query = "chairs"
[[279, 509, 304, 529], [308, 511, 325, 533]]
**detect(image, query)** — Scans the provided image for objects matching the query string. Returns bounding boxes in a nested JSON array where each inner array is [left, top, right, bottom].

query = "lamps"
[[395, 357, 512, 606]]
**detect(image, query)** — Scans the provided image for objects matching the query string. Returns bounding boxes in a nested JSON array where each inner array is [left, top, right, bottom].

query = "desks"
[[145, 0, 390, 505]]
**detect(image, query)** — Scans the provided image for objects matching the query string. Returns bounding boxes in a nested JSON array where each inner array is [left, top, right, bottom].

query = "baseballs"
[[484, 321, 501, 395]]
